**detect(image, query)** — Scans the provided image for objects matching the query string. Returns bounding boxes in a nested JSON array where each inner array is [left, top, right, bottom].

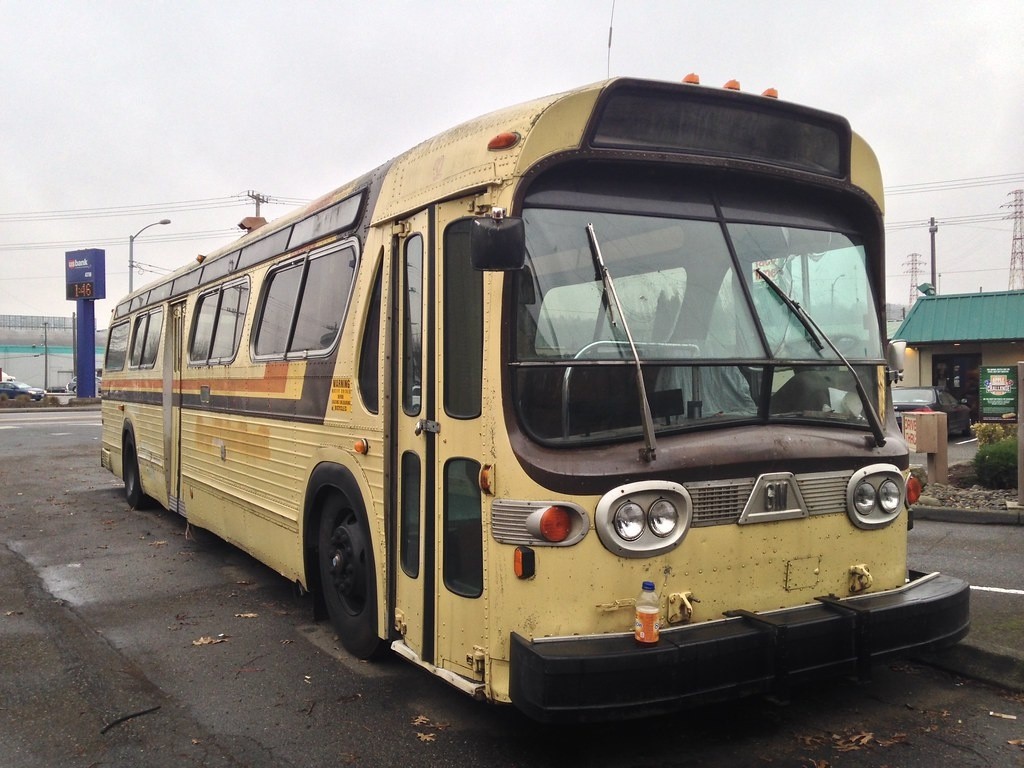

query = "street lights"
[[128, 219, 172, 293], [42, 322, 49, 390]]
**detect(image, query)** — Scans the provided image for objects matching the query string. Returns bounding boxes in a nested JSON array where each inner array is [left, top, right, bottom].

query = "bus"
[[96, 70, 973, 720]]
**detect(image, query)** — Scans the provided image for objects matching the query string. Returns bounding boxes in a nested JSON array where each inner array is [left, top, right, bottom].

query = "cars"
[[68, 376, 102, 394], [888, 382, 973, 440], [0, 381, 47, 401]]
